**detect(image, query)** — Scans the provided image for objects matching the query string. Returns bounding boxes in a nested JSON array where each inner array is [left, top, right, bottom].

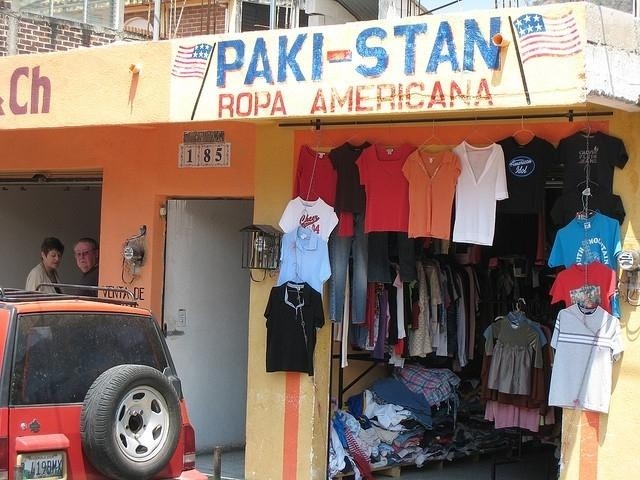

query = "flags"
[[513, 10, 582, 63], [170, 43, 214, 78]]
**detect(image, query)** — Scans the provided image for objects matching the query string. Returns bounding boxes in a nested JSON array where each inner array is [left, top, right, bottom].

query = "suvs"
[[0, 283, 209, 480]]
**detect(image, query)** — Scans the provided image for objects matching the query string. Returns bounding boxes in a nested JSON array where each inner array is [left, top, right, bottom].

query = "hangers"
[[344, 115, 599, 158], [513, 297, 528, 318], [286, 270, 308, 296], [576, 160, 598, 226]]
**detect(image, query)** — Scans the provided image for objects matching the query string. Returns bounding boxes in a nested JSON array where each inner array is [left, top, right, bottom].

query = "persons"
[[26, 237, 65, 294], [74, 237, 99, 297]]
